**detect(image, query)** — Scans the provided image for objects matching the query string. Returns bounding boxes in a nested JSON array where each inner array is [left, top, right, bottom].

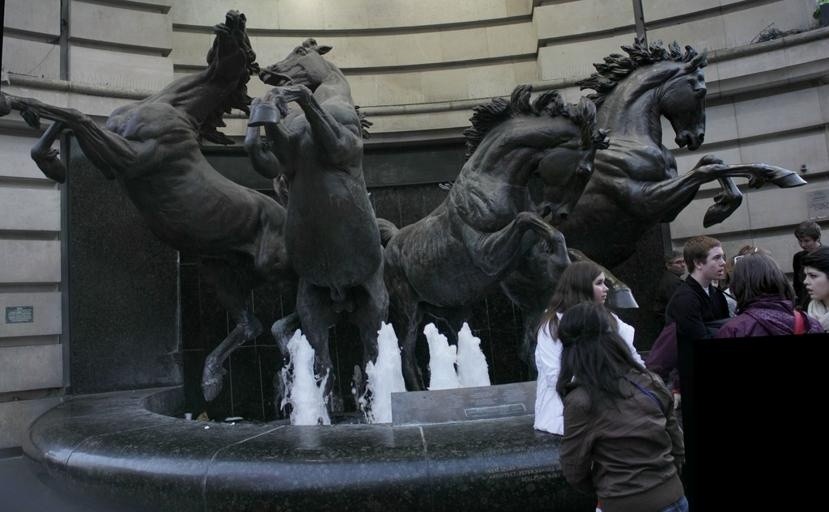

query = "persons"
[[647, 218, 829, 420], [533, 258, 647, 437], [557, 299, 691, 511]]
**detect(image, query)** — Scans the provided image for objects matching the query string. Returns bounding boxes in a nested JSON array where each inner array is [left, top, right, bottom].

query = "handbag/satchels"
[[644, 318, 732, 375]]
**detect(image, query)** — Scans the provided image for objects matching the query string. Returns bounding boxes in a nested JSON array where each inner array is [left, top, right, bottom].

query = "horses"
[[468, 35, 808, 384], [377, 83, 639, 392], [245, 36, 391, 423], [1, 9, 300, 405]]
